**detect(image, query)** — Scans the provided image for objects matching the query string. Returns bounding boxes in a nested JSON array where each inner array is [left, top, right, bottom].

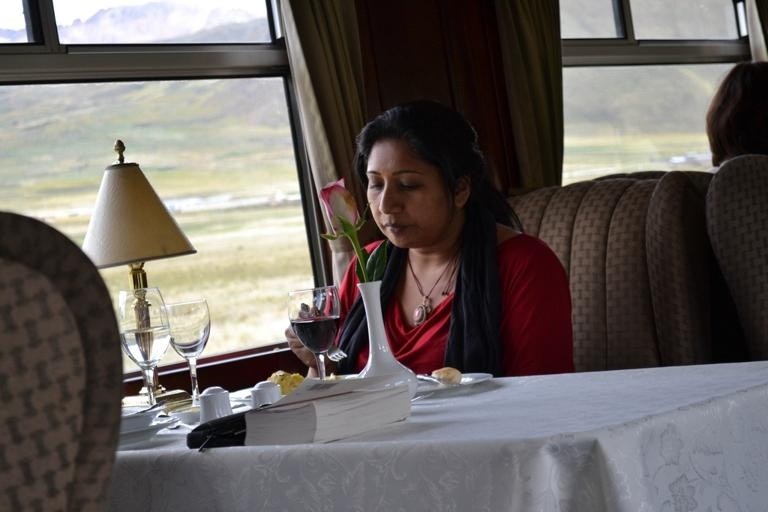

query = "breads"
[[431, 367, 461, 385]]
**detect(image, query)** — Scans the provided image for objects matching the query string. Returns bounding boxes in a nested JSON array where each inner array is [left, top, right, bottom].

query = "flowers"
[[311, 180, 387, 282]]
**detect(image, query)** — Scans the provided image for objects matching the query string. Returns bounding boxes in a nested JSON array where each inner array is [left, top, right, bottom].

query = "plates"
[[117, 406, 181, 447], [414, 372, 494, 396]]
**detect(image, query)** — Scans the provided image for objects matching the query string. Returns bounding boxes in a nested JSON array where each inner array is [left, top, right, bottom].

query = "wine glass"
[[117, 286, 172, 406], [160, 298, 211, 408], [287, 284, 342, 382]]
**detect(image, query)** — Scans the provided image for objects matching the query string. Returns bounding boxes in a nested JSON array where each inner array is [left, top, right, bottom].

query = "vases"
[[358, 280, 417, 397]]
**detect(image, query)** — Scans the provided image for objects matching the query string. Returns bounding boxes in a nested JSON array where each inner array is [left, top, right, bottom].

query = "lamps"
[[80, 139, 198, 410]]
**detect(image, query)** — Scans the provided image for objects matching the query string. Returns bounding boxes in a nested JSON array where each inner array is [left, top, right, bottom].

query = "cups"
[[198, 385, 234, 426], [250, 378, 283, 412]]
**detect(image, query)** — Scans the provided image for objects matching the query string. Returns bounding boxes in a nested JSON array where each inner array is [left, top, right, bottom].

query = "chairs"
[[504, 171, 710, 371]]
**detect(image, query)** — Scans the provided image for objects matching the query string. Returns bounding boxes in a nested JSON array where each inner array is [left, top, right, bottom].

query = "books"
[[186, 373, 424, 450]]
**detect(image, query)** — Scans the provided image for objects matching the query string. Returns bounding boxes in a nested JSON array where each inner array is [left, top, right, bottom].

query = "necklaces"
[[406, 260, 456, 323]]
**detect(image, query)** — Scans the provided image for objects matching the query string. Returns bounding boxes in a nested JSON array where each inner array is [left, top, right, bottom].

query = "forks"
[[327, 345, 348, 363]]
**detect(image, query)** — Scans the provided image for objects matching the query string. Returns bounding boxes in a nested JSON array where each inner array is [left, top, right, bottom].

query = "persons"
[[704, 59, 767, 173], [275, 96, 574, 378]]
